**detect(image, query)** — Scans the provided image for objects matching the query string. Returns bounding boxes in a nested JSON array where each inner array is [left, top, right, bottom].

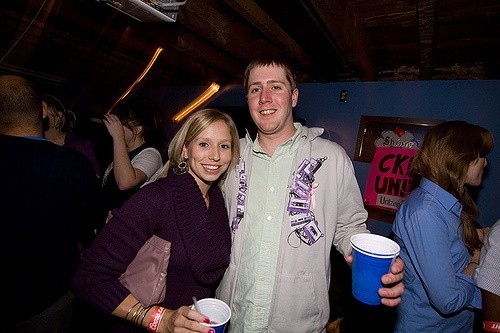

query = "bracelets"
[[125, 300, 166, 333]]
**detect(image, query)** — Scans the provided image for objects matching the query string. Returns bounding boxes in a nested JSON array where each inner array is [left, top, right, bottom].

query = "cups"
[[350, 232, 402, 306], [191, 297, 233, 333]]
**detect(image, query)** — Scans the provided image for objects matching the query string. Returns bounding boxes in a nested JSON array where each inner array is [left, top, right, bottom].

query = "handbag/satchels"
[[104, 211, 172, 309]]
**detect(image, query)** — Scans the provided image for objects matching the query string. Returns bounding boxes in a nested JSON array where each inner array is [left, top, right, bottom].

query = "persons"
[[0, 75, 104, 333], [392, 120, 500, 333], [73, 108, 240, 333], [195, 56, 404, 333], [99, 106, 163, 221]]
